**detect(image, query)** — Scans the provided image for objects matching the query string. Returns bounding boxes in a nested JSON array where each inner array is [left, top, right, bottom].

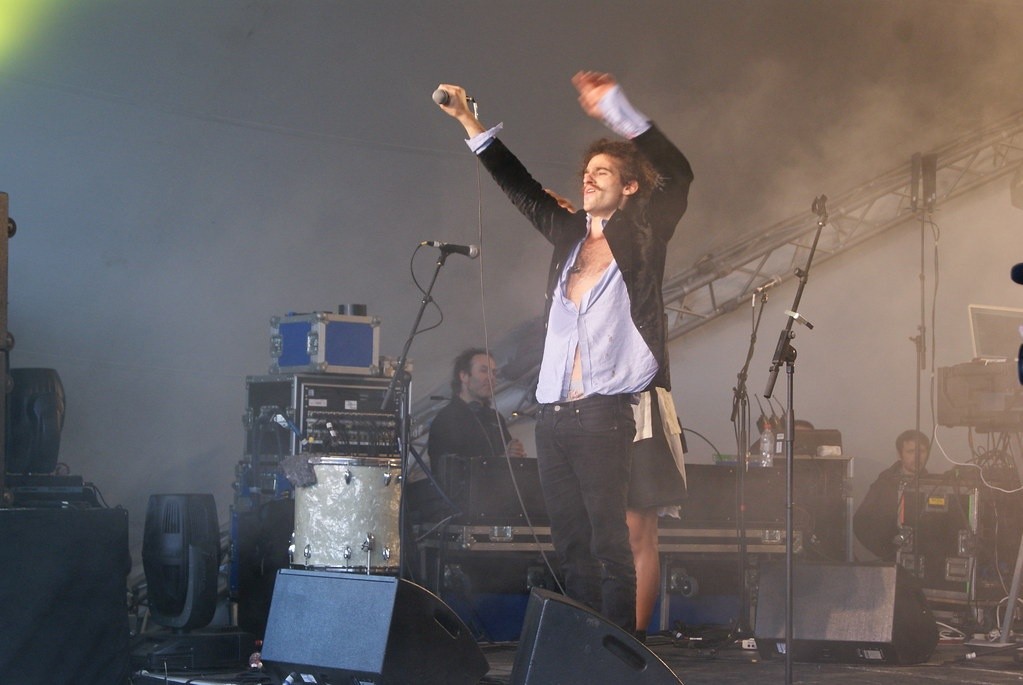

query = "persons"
[[851, 430, 954, 568], [542, 189, 687, 643], [426, 342, 526, 468], [431, 72, 696, 655]]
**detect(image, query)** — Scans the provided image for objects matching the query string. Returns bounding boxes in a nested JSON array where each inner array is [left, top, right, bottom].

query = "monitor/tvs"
[[775, 429, 843, 457], [968, 304, 1023, 361]]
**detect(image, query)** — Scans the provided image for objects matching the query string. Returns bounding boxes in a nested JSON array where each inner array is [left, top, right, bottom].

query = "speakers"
[[750, 557, 940, 668], [260, 567, 491, 685], [510, 587, 684, 685]]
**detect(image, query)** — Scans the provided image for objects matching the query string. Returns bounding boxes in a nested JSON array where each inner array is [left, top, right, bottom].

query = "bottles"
[[758, 424, 775, 468]]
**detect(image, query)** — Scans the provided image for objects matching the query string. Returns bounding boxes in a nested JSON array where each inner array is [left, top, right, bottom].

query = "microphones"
[[426, 240, 481, 259], [432, 88, 475, 106], [754, 274, 782, 295], [430, 395, 454, 401]]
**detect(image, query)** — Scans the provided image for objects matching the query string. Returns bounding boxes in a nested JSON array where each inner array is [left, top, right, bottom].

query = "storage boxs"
[[269, 312, 381, 375], [233, 463, 404, 578]]
